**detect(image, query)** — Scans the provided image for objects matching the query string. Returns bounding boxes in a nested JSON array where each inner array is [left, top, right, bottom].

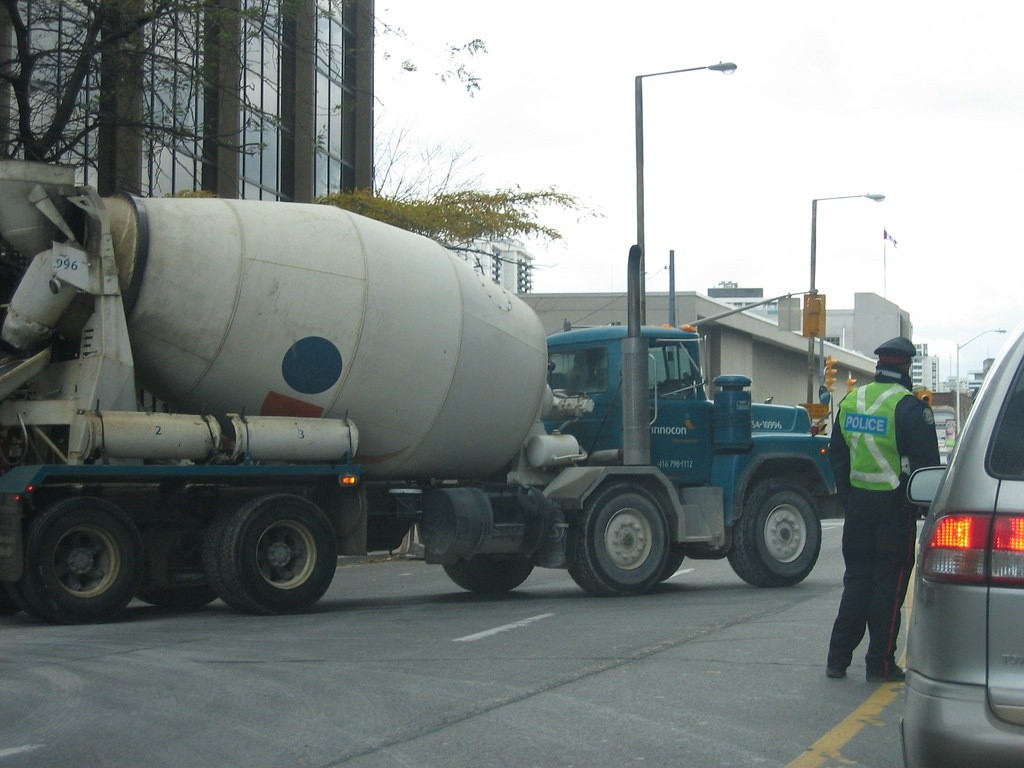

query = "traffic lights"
[[918, 389, 931, 407], [847, 377, 857, 393], [825, 355, 838, 393], [957, 328, 1005, 436]]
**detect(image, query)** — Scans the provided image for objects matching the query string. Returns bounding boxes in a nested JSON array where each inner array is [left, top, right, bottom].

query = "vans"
[[931, 405, 959, 456], [901, 333, 1024, 768]]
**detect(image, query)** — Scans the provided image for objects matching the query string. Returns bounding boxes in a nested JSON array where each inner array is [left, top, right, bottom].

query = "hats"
[[874, 337, 917, 365]]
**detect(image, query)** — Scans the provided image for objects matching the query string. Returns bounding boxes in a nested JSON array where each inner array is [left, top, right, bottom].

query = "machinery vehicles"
[[0, 156, 848, 623]]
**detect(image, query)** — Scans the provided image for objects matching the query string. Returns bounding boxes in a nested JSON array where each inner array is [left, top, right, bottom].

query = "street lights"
[[807, 194, 885, 432], [634, 63, 738, 327]]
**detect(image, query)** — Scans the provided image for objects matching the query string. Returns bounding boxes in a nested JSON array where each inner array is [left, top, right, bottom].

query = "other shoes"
[[825, 667, 846, 678], [866, 666, 905, 682]]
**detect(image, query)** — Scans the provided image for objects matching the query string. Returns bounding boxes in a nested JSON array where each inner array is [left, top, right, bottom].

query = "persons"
[[825, 337, 942, 682]]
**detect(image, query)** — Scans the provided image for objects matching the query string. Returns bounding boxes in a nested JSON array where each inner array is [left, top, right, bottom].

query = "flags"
[[884, 229, 898, 248]]
